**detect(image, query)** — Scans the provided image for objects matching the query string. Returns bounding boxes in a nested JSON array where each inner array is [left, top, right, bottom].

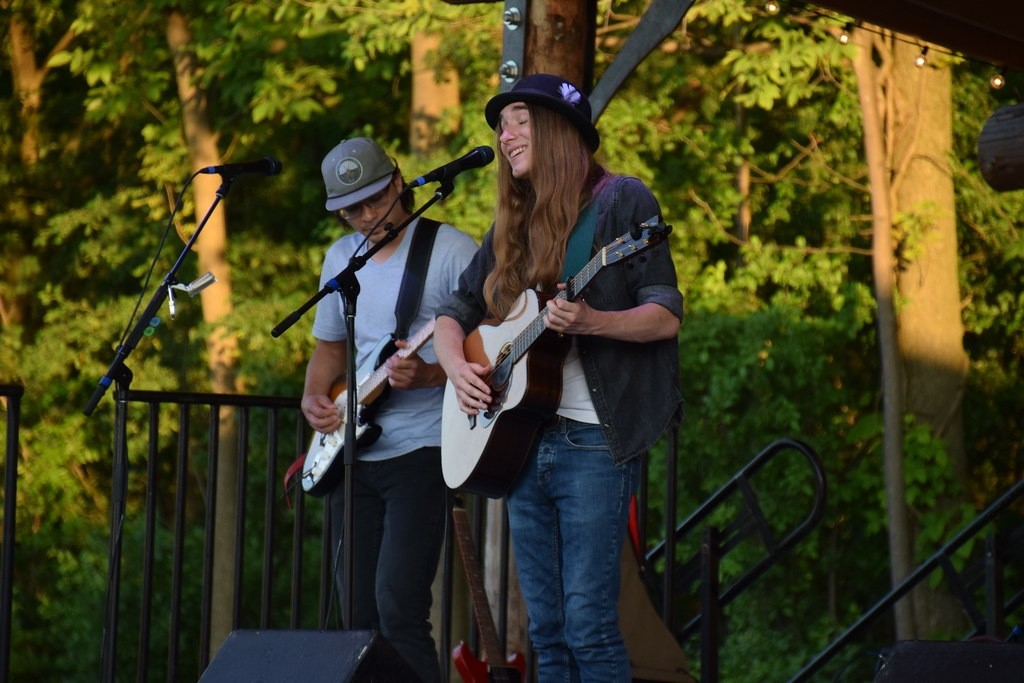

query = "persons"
[[431, 74, 684, 683], [300, 137, 480, 683]]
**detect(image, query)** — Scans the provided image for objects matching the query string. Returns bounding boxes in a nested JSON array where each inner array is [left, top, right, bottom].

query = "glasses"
[[338, 177, 392, 221]]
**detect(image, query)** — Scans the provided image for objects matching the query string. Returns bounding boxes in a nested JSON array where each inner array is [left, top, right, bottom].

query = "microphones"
[[410, 146, 495, 188], [200, 156, 282, 175]]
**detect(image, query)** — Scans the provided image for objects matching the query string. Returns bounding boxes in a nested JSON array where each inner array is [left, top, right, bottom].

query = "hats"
[[321, 137, 398, 212], [483, 75, 600, 153]]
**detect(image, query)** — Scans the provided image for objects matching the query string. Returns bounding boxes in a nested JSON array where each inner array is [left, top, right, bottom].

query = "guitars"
[[452, 490, 527, 683], [439, 213, 675, 499], [301, 316, 435, 499]]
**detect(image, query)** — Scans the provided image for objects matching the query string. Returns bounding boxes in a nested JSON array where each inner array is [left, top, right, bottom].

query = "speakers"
[[198, 629, 424, 683], [874, 640, 1024, 683]]
